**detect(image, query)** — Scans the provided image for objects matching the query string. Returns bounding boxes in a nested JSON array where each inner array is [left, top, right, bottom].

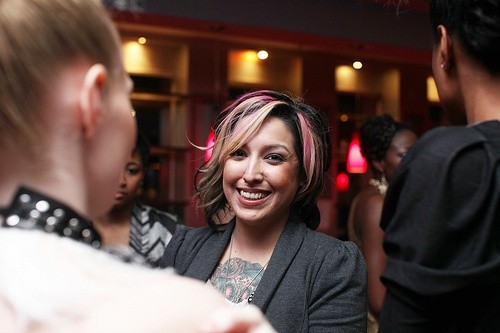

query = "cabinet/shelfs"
[[108, 8, 448, 229]]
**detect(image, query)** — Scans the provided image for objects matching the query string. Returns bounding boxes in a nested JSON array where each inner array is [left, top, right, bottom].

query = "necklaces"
[[224, 234, 270, 303]]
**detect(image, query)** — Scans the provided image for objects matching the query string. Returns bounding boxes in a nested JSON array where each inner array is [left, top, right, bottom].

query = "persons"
[[92, 127, 178, 260], [380, 0, 500, 333], [0, 0, 277, 333], [156, 90, 367, 333], [348, 113, 419, 333]]
